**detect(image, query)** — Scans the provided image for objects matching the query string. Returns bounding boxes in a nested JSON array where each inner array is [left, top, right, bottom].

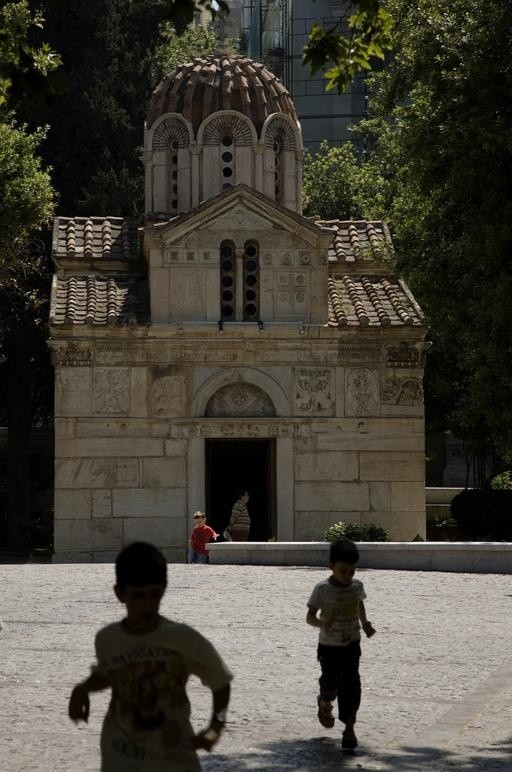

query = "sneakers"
[[317, 694, 336, 728], [342, 730, 360, 748]]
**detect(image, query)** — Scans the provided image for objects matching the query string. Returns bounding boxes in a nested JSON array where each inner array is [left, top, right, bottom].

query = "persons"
[[303, 534, 378, 749], [225, 488, 259, 535], [184, 511, 218, 557], [69, 540, 230, 771]]
